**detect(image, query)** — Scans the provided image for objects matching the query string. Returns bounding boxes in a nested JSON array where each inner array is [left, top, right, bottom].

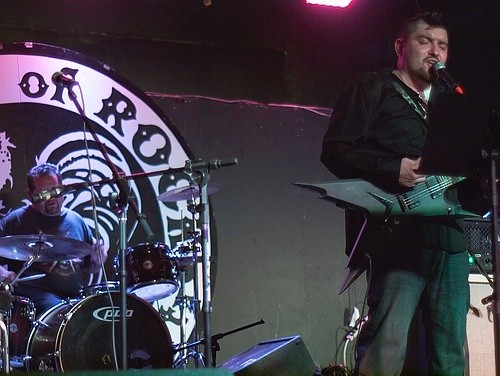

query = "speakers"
[[216, 334, 319, 376], [464, 217, 499, 376]]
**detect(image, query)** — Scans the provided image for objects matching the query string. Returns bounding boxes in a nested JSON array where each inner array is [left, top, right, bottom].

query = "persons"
[[0, 162, 109, 318], [320, 9, 472, 376]]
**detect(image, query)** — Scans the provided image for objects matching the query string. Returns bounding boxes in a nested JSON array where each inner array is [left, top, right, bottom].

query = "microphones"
[[30, 187, 68, 204], [431, 62, 464, 96], [51, 72, 79, 86], [481, 294, 494, 305]]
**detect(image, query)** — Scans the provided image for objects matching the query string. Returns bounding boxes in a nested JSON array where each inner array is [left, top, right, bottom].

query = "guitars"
[[286, 155, 484, 298]]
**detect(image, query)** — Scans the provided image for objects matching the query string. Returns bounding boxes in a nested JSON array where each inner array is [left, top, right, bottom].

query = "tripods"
[[173, 195, 205, 368]]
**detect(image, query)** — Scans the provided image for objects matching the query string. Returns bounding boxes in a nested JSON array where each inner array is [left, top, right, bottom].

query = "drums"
[[0, 294, 37, 368], [25, 289, 177, 375], [110, 239, 182, 304], [79, 281, 127, 298]]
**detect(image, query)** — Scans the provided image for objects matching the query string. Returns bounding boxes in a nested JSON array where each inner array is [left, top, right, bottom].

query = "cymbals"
[[0, 234, 94, 265], [156, 182, 227, 204]]
